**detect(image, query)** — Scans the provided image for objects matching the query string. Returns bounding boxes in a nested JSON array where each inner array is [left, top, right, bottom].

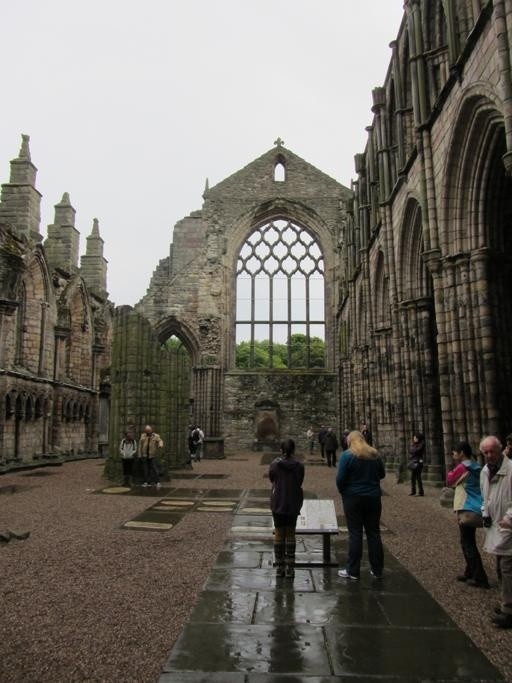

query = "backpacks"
[[192, 429, 201, 441]]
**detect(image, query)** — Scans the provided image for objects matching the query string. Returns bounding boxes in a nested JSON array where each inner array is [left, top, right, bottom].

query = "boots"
[[286, 542, 296, 578], [274, 543, 285, 578]]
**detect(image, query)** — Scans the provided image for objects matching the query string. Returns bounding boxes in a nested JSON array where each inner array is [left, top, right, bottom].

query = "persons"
[[305, 424, 316, 455], [137, 423, 165, 489], [406, 433, 426, 496], [267, 438, 305, 580], [446, 439, 492, 589], [479, 435, 512, 629], [501, 432, 512, 459], [191, 423, 205, 462], [334, 428, 387, 581], [322, 427, 339, 467], [119, 429, 139, 487], [360, 424, 373, 447], [318, 424, 327, 457], [341, 427, 350, 451]]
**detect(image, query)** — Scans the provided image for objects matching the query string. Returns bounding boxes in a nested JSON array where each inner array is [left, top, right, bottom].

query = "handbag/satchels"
[[407, 460, 424, 471]]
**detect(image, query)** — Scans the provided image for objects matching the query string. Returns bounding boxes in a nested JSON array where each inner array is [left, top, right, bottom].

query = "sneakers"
[[157, 483, 162, 487], [338, 569, 358, 580], [142, 483, 149, 487], [456, 574, 488, 587], [489, 609, 512, 627]]
